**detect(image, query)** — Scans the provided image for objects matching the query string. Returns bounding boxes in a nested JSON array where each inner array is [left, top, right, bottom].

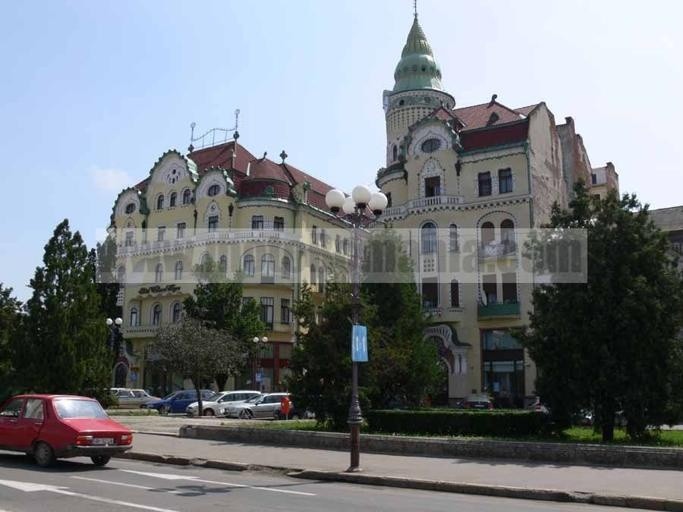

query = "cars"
[[458, 395, 494, 409], [0, 394, 135, 467], [528, 393, 551, 414], [577, 408, 597, 426]]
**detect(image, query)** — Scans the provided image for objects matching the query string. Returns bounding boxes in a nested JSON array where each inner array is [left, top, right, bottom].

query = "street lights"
[[322, 183, 391, 475], [253, 335, 269, 391], [105, 316, 122, 385]]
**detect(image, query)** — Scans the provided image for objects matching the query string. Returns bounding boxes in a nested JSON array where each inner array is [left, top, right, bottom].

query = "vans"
[[105, 388, 296, 419]]
